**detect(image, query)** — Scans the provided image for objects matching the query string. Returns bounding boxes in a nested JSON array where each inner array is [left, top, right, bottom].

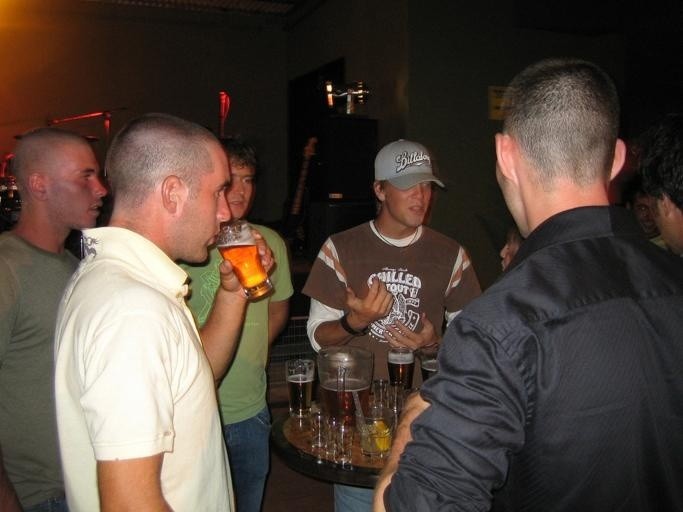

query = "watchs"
[[340, 315, 370, 338]]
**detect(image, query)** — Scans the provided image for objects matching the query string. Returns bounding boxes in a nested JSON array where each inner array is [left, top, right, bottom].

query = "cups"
[[311, 414, 329, 449], [386, 382, 405, 413], [214, 223, 278, 304], [285, 361, 315, 420], [372, 378, 389, 411], [318, 345, 376, 425], [356, 407, 397, 459], [420, 350, 441, 383], [326, 417, 347, 455], [388, 347, 415, 390], [336, 426, 354, 458]]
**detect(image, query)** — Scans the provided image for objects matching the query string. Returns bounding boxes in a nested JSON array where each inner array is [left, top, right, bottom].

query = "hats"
[[375, 139, 445, 190]]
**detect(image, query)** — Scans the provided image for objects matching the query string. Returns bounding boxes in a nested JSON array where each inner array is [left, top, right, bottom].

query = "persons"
[[177, 136, 295, 512], [53, 112, 278, 512], [1, 130, 106, 512], [625, 172, 667, 253], [500, 224, 524, 274], [302, 139, 481, 512], [372, 58, 683, 512], [640, 115, 683, 256]]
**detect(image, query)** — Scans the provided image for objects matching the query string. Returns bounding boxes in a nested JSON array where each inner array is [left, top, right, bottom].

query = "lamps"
[[326, 82, 370, 114]]
